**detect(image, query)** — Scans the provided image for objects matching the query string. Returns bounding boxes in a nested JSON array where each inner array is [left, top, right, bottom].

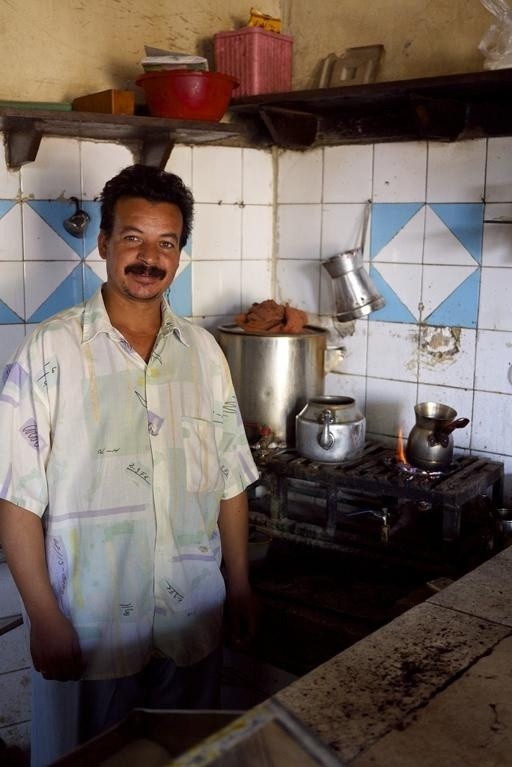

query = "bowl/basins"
[[135, 70, 241, 122]]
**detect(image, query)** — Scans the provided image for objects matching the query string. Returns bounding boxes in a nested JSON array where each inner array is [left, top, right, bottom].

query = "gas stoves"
[[240, 435, 505, 545]]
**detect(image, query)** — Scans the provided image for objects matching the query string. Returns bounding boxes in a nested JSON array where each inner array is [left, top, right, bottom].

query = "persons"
[[0, 165, 261, 767]]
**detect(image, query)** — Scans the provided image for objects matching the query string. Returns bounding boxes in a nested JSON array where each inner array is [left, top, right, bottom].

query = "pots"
[[406, 402, 468, 471], [218, 321, 347, 451]]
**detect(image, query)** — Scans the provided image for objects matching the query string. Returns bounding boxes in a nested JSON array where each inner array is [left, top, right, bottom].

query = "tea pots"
[[294, 394, 368, 465]]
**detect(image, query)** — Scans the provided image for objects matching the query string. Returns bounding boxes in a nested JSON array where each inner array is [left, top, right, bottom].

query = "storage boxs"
[[213, 27, 294, 98]]
